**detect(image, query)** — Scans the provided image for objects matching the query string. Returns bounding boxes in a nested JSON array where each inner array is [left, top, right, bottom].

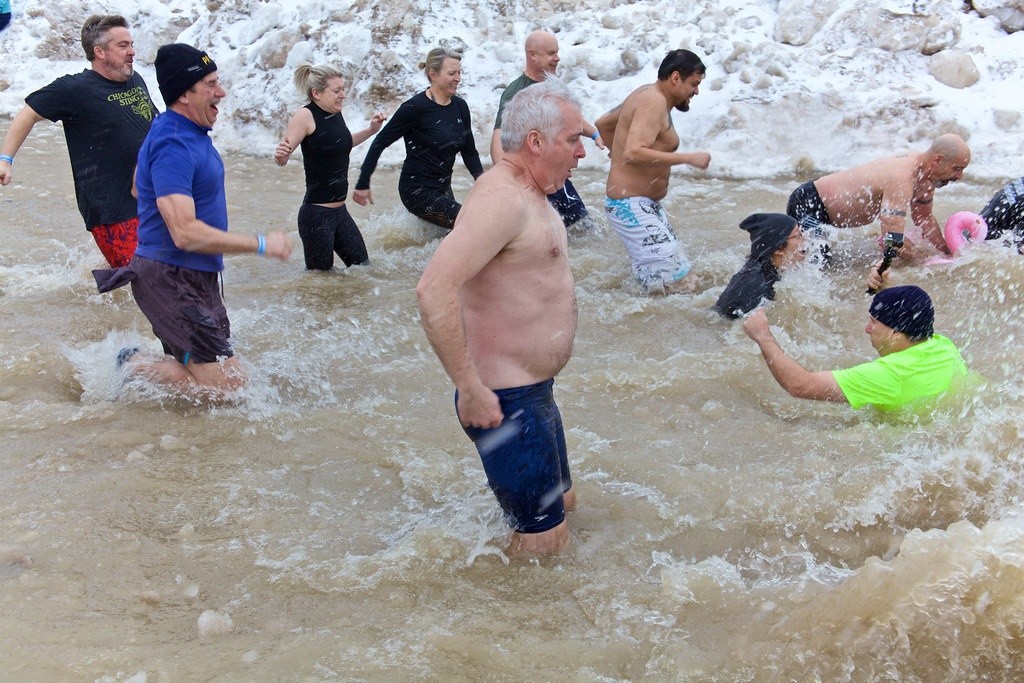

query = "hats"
[[869, 286, 934, 335], [155, 43, 218, 105], [738, 214, 797, 258]]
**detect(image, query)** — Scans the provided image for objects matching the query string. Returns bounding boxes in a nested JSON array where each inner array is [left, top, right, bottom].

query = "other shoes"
[[116, 348, 138, 384]]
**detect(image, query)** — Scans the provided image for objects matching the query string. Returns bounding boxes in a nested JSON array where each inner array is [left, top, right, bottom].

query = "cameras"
[[883, 231, 904, 252]]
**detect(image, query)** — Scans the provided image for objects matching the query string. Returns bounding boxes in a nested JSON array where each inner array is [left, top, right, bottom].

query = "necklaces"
[[428, 88, 438, 103]]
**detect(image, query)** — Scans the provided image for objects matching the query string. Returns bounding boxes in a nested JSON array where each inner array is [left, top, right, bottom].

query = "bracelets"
[[943, 248, 950, 256], [255, 232, 269, 257], [0, 153, 13, 165], [591, 131, 601, 140]]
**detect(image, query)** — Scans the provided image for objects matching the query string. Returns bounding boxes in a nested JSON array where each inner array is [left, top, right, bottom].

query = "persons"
[[353, 48, 486, 230], [785, 132, 971, 283], [0, 0, 12, 30], [744, 265, 976, 426], [273, 65, 389, 275], [490, 31, 613, 240], [414, 78, 587, 564], [974, 176, 1024, 257], [595, 48, 712, 295], [0, 14, 166, 286], [713, 211, 810, 322], [108, 42, 294, 413]]
